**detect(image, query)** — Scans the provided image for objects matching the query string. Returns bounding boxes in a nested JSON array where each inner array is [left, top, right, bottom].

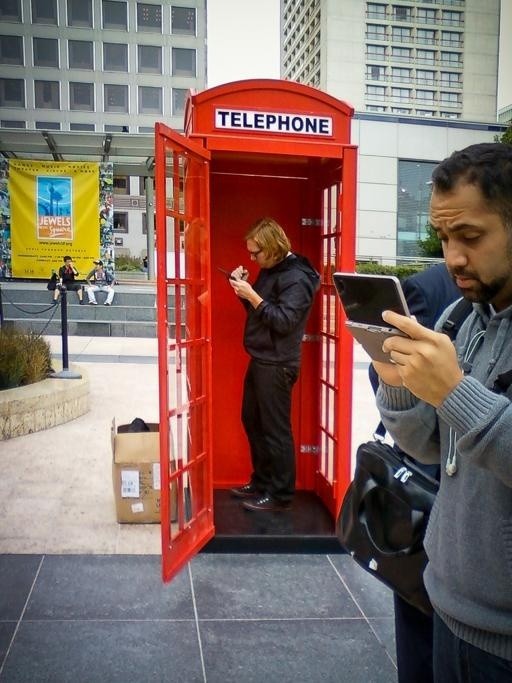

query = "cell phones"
[[228, 273, 236, 281]]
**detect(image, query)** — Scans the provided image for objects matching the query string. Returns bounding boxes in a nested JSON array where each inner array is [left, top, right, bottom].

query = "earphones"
[[445, 458, 454, 476], [449, 455, 457, 473]]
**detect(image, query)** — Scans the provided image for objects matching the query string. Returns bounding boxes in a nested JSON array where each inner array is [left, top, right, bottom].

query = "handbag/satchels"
[[335, 441, 439, 613], [47, 270, 57, 292]]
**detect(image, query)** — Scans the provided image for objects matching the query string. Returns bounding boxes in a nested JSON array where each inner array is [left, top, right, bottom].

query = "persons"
[[369, 139, 510, 682], [85, 259, 117, 305], [49, 255, 84, 304], [368, 258, 474, 683], [142, 255, 148, 278], [228, 215, 322, 512]]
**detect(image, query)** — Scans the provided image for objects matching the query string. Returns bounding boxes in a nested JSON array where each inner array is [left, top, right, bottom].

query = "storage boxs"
[[108, 415, 178, 525]]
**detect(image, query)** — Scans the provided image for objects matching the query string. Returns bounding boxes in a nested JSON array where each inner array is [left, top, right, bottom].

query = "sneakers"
[[103, 302, 111, 306], [228, 482, 294, 510], [79, 300, 98, 305], [50, 300, 59, 304]]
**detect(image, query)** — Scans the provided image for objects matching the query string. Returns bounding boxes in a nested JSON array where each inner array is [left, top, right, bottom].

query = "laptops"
[[333, 272, 413, 364]]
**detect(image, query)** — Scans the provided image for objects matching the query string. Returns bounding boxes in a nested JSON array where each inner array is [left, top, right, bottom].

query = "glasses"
[[250, 248, 264, 253]]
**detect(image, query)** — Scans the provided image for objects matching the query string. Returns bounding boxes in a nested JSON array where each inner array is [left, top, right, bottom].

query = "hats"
[[93, 258, 104, 266]]
